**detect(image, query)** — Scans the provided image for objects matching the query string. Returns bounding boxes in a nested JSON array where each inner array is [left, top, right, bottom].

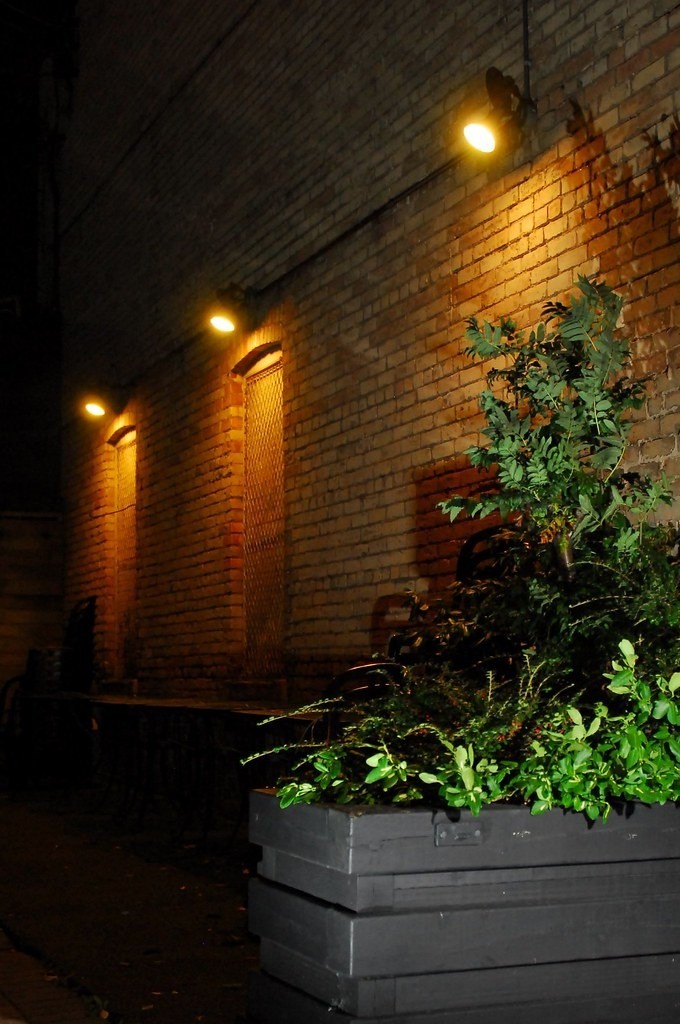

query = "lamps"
[[463, 66, 524, 154], [210, 282, 245, 332], [85, 382, 125, 418]]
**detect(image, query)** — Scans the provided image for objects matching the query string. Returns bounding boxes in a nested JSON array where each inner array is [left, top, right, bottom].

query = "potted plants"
[[239, 273, 680, 1024]]
